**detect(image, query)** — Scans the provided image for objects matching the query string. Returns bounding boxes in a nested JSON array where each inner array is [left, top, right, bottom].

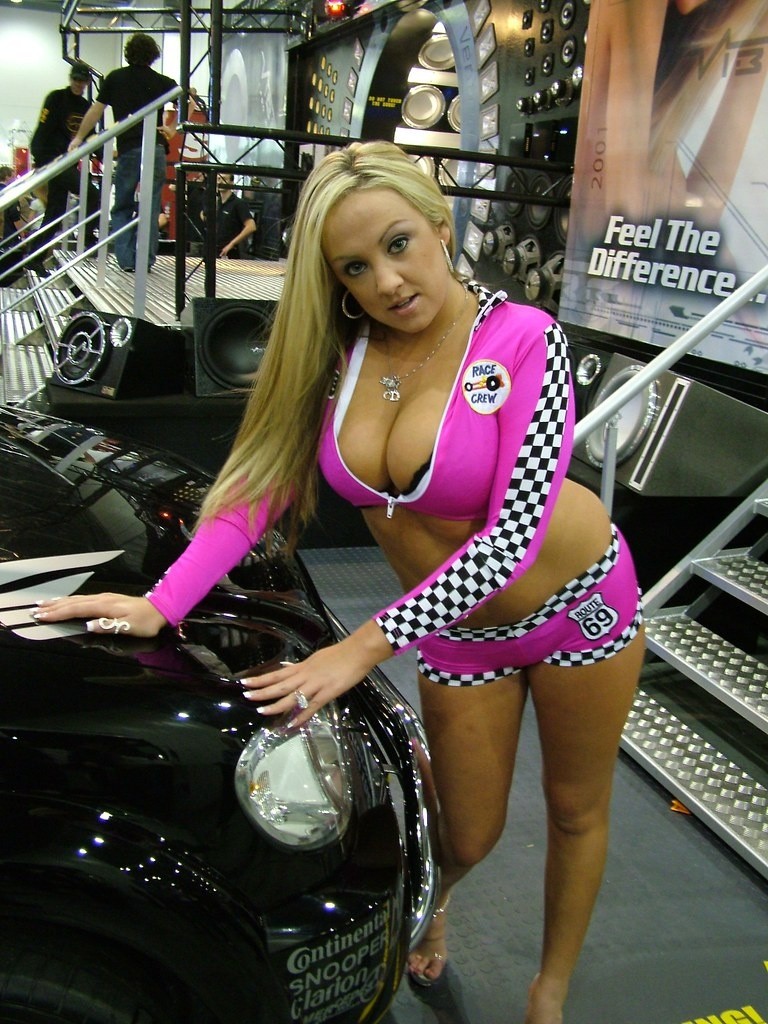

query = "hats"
[[70, 64, 91, 81]]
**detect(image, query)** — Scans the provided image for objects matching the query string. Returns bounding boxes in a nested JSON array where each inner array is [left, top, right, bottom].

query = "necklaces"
[[379, 283, 468, 402]]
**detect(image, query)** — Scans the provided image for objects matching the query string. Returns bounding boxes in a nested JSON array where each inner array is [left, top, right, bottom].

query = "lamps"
[[401, 35, 463, 187]]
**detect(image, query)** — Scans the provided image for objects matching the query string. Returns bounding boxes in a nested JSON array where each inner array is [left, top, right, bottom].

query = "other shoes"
[[148, 267, 152, 272], [22, 254, 51, 278], [85, 233, 98, 248], [124, 267, 133, 272]]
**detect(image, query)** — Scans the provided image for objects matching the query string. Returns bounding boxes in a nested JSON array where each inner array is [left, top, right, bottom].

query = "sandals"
[[408, 894, 451, 988]]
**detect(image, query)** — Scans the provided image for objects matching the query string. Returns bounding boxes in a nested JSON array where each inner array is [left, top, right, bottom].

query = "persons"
[[0, 160, 256, 292], [24, 60, 103, 279], [29, 140, 646, 1024], [67, 34, 196, 274], [569, 0, 767, 281]]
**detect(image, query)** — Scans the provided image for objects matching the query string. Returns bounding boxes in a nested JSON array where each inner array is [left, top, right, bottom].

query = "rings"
[[294, 691, 308, 709], [99, 617, 131, 634]]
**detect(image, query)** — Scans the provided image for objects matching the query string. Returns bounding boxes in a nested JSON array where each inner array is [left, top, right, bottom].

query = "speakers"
[[50, 307, 186, 402], [180, 296, 280, 397], [567, 340, 768, 498]]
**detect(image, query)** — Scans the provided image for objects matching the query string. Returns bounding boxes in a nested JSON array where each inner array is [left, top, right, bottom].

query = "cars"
[[1, 404, 442, 1024]]
